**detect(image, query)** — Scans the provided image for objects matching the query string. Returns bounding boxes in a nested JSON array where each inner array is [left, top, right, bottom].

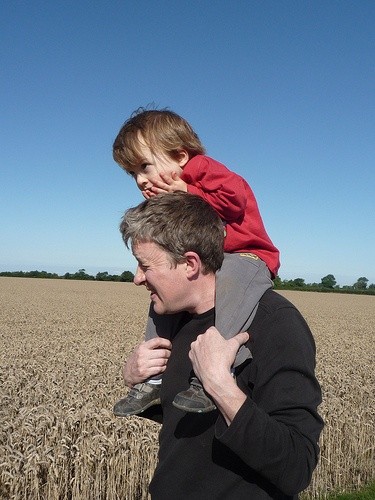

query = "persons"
[[120, 192, 322, 500], [112, 108, 279, 417]]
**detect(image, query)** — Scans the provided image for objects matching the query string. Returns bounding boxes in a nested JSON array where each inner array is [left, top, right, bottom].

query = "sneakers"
[[172, 375, 237, 413], [113, 383, 162, 417]]
